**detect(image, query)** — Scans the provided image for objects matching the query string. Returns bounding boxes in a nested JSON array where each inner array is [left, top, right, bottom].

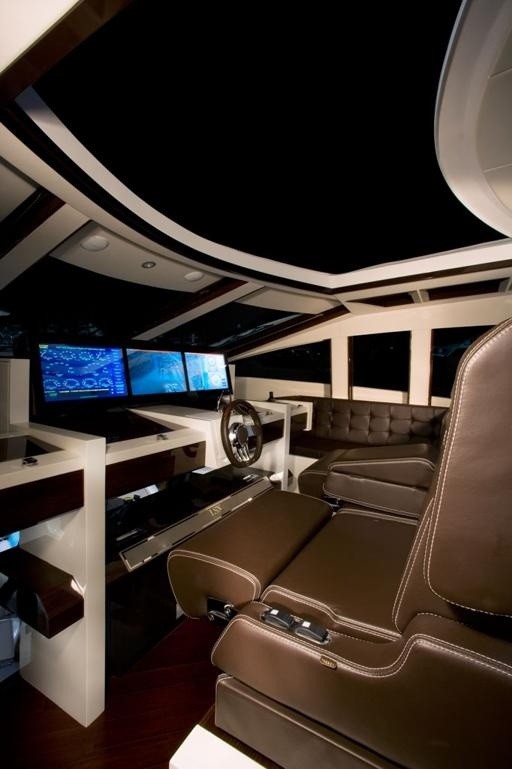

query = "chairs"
[[174, 326, 512, 753]]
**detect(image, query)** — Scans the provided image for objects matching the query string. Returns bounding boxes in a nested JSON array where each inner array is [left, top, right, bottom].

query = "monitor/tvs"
[[182, 345, 233, 398], [31, 331, 130, 410], [124, 340, 189, 403]]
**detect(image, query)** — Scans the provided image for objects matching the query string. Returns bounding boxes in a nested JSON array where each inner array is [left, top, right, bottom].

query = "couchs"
[[279, 393, 453, 487]]
